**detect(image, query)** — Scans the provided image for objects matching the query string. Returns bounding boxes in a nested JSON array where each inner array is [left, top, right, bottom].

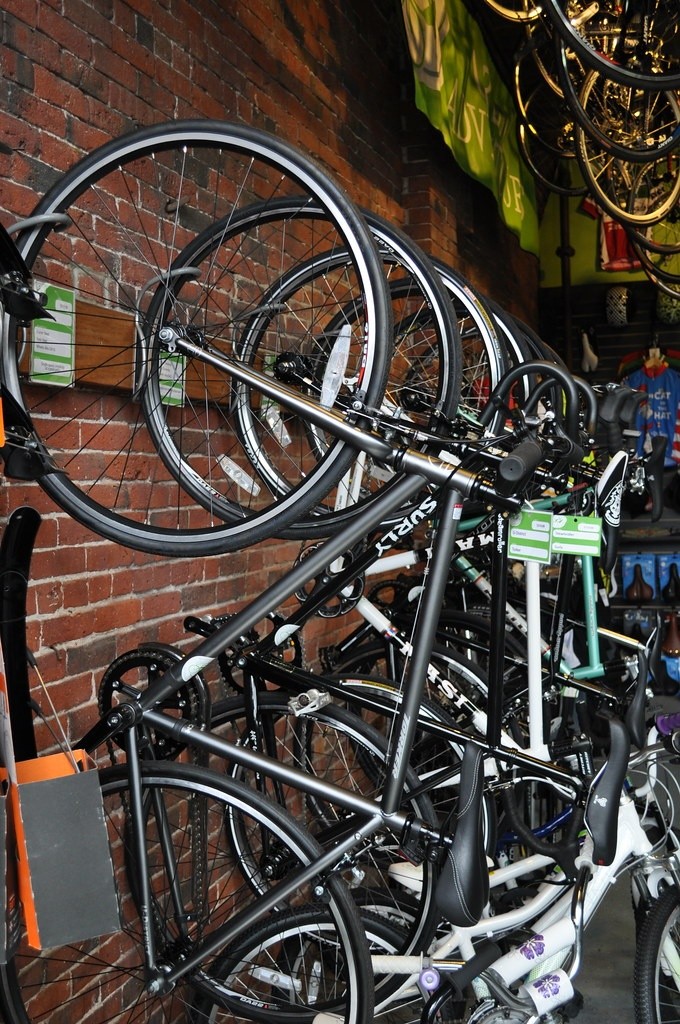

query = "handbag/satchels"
[[0, 642, 121, 953]]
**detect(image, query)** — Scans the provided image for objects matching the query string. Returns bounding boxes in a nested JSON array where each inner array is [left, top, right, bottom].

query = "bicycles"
[[1, 119, 680, 1024], [464, 1, 680, 305]]
